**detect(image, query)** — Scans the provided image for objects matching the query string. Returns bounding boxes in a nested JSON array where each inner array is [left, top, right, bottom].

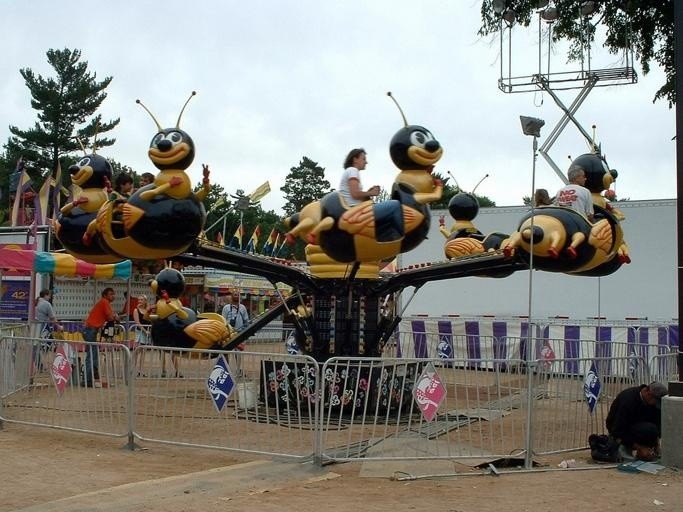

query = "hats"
[[649, 381, 667, 398]]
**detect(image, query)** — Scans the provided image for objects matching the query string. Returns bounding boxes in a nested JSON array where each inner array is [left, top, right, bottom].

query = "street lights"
[[518, 114, 544, 469], [233, 187, 250, 251]]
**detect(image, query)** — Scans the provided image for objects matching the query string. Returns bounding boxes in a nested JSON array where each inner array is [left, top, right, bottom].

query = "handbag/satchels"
[[589, 433, 615, 462]]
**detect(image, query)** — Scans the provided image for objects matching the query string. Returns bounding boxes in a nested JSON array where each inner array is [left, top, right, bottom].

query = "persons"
[[81, 288, 115, 388], [222, 291, 249, 377], [132, 294, 151, 377], [139, 173, 155, 188], [339, 148, 380, 208], [556, 165, 594, 222], [159, 351, 184, 378], [203, 292, 215, 313], [108, 173, 133, 201], [534, 189, 554, 207], [605, 381, 668, 462], [28, 290, 64, 374], [121, 292, 139, 321]]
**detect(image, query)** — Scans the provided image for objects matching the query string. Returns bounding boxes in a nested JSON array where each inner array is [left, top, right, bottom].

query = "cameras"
[[230, 320, 235, 326]]
